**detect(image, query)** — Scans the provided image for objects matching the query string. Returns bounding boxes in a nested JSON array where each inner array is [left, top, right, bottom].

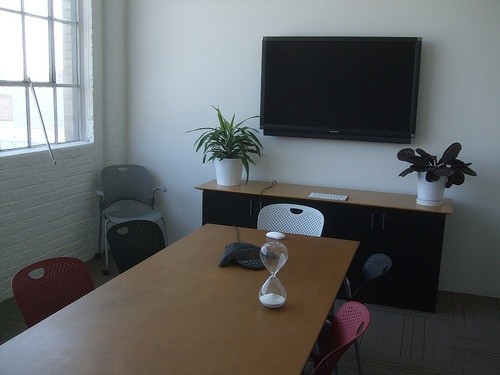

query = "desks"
[[1, 223, 361, 374]]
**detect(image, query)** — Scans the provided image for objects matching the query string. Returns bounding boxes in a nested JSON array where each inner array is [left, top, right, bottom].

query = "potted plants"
[[185, 105, 264, 186], [398, 142, 478, 207]]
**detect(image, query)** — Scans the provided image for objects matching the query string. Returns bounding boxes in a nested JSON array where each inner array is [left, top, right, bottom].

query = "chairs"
[[107, 220, 167, 276], [343, 253, 392, 298], [94, 165, 169, 274], [307, 302, 371, 375], [256, 204, 324, 238], [12, 256, 96, 327]]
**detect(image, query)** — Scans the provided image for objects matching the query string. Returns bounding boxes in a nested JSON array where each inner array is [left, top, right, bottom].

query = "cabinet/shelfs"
[[195, 178, 456, 313]]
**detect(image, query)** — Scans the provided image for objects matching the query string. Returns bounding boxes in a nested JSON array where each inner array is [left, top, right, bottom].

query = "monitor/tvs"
[[258, 36, 421, 144]]
